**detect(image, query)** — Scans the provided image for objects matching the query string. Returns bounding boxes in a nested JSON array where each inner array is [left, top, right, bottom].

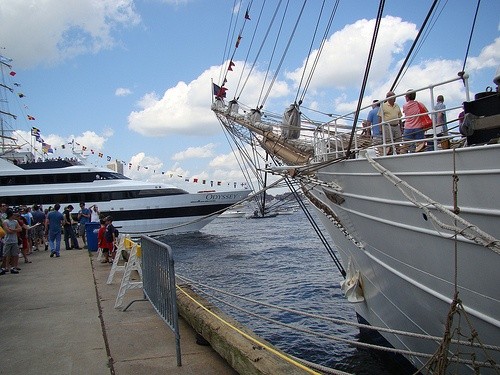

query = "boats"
[[216, 211, 247, 218], [246, 205, 295, 219], [209, 0, 500, 375]]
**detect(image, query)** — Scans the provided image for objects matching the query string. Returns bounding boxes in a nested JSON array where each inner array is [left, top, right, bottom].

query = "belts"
[[385, 124, 399, 126]]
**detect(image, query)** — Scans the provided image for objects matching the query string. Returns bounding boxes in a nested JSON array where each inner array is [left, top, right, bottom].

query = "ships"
[[0, 44, 254, 240]]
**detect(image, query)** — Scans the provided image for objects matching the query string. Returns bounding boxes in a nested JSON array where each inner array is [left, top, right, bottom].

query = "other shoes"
[[0, 268, 6, 274], [45, 245, 48, 251], [11, 269, 19, 274], [76, 248, 82, 249], [25, 260, 32, 263], [50, 252, 56, 257], [6, 269, 9, 272], [66, 248, 73, 250], [56, 256, 59, 257]]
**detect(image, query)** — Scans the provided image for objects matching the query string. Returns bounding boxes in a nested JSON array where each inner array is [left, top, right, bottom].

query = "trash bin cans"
[[85, 222, 101, 251]]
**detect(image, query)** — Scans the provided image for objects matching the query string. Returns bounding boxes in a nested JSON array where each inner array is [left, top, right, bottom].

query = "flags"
[[31, 127, 53, 153], [62, 143, 72, 149], [76, 142, 164, 174], [170, 174, 244, 188], [213, 83, 226, 98], [10, 72, 26, 98], [27, 115, 35, 120]]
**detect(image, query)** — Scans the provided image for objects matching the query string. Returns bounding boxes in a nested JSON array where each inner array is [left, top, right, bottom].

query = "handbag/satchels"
[[111, 224, 119, 237], [416, 101, 432, 128]]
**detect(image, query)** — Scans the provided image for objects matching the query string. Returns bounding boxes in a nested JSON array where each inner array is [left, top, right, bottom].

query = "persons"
[[63, 202, 83, 250], [13, 156, 77, 165], [376, 92, 402, 155], [433, 95, 450, 150], [0, 204, 54, 274], [400, 90, 428, 154], [98, 216, 119, 263], [78, 202, 90, 247], [89, 204, 100, 222], [367, 100, 381, 153], [361, 120, 371, 146], [46, 204, 65, 257]]
